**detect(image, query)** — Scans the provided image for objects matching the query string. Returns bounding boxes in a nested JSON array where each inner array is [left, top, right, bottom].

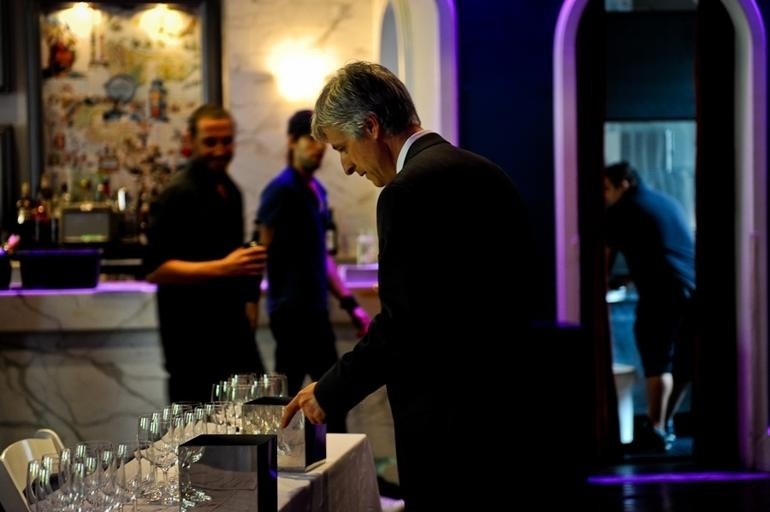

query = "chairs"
[[0, 428, 66, 512]]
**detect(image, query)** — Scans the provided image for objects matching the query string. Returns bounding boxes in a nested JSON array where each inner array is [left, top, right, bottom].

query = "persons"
[[603, 161, 698, 453], [245, 110, 372, 499], [142, 103, 270, 413], [278, 62, 572, 512]]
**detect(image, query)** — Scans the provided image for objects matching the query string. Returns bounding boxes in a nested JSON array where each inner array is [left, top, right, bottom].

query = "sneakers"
[[632, 418, 676, 449]]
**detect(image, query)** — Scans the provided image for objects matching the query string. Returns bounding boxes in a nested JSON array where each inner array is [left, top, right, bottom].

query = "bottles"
[[245, 220, 264, 285], [325, 209, 337, 255], [15, 174, 149, 247], [356, 229, 373, 265]]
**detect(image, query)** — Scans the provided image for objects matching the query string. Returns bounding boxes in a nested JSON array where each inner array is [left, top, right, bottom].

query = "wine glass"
[[27, 373, 286, 512]]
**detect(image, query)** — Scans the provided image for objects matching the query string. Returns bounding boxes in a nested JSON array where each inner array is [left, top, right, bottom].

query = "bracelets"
[[340, 295, 359, 314]]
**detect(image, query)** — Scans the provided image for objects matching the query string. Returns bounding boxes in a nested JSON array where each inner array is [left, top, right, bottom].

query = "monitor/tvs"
[[59, 207, 113, 248]]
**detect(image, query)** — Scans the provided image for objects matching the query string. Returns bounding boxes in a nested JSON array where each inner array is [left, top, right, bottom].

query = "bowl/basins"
[[0, 249, 103, 290]]
